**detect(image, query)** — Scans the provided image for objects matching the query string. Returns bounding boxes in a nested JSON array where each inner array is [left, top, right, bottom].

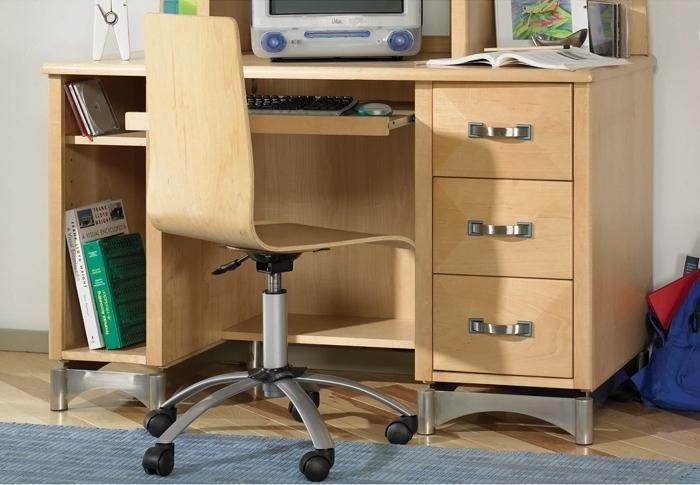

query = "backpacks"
[[616, 270, 700, 414]]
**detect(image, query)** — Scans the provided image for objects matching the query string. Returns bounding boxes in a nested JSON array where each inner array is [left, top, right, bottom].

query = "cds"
[[83, 82, 113, 131]]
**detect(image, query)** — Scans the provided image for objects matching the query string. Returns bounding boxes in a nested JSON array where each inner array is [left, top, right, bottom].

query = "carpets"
[[1, 416, 700, 485]]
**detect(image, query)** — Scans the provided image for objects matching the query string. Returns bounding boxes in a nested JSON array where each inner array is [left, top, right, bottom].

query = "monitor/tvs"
[[250, 0, 423, 58]]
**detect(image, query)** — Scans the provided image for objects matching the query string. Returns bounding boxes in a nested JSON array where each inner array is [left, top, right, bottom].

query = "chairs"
[[131, 9, 423, 485]]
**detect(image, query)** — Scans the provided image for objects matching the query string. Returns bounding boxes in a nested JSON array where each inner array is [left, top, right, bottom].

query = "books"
[[65, 199, 129, 350], [83, 233, 146, 350], [425, 46, 630, 71]]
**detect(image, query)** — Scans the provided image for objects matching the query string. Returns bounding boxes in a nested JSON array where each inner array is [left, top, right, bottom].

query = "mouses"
[[359, 102, 392, 116]]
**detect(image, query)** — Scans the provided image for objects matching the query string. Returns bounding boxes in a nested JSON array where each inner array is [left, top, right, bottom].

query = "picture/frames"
[[488, 0, 595, 56]]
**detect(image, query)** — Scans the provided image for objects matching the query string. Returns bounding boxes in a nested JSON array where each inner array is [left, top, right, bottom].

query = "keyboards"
[[245, 93, 359, 116]]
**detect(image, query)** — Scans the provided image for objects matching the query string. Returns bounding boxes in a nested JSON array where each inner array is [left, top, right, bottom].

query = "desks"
[[37, 2, 660, 448]]
